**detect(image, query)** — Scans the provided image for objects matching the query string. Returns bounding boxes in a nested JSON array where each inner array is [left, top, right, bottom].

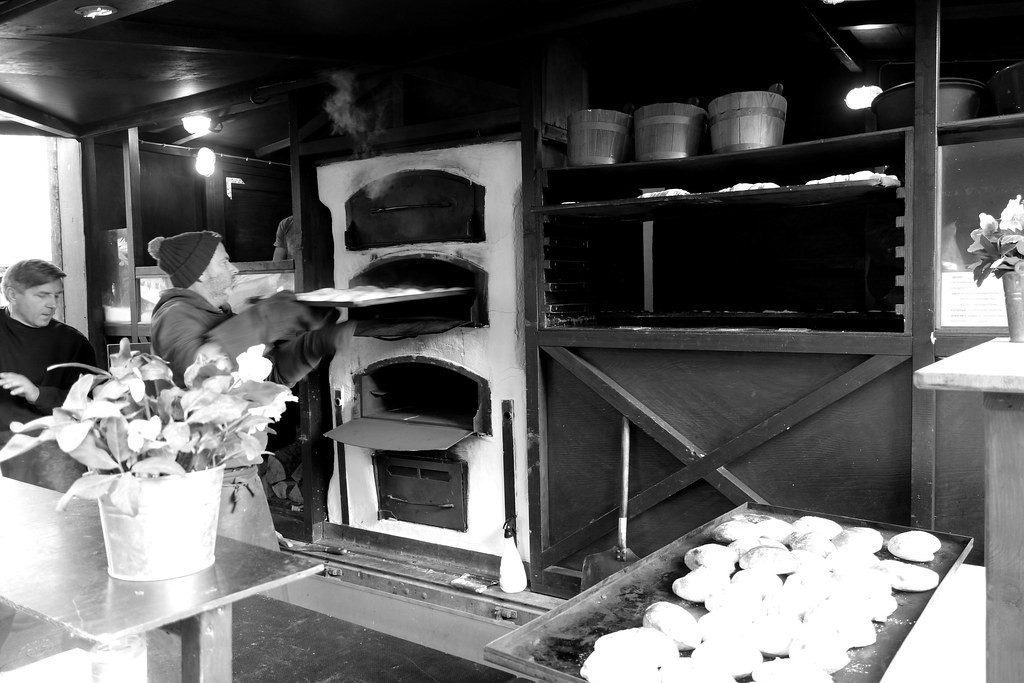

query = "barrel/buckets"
[[566, 90, 788, 167]]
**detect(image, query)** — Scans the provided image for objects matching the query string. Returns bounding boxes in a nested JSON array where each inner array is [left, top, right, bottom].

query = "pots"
[[987, 61, 1024, 115]]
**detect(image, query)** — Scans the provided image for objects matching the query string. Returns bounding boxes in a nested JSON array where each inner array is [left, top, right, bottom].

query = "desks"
[[0, 475, 325, 680]]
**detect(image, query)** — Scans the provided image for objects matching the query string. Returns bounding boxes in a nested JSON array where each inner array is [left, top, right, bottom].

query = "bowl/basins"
[[871, 77, 986, 130]]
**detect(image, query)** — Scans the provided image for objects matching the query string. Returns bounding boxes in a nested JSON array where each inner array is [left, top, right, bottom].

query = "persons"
[[273, 215, 298, 262], [148, 232, 357, 552], [0, 258, 99, 434]]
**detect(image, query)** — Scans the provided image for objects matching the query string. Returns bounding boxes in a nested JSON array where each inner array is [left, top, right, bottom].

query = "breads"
[[579, 516, 942, 683]]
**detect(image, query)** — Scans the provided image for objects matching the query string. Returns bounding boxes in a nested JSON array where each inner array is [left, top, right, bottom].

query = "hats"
[[147, 232, 224, 290]]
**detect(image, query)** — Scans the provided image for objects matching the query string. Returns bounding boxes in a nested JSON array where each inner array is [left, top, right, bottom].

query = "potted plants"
[[967, 193, 1023, 343], [0, 337, 298, 581]]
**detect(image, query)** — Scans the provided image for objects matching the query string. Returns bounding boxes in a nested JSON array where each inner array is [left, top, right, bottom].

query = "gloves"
[[206, 288, 326, 359], [300, 309, 358, 359]]
